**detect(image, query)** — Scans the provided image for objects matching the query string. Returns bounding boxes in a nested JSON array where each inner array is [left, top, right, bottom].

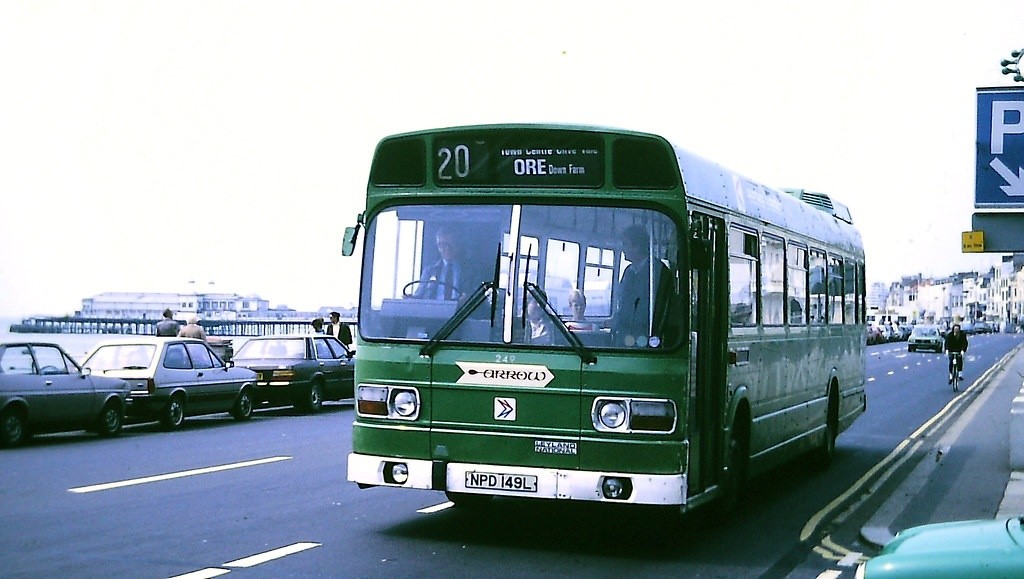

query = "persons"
[[614, 224, 671, 346], [311, 312, 355, 346], [155, 308, 208, 343], [521, 288, 601, 346], [943, 325, 968, 384], [413, 225, 473, 300]]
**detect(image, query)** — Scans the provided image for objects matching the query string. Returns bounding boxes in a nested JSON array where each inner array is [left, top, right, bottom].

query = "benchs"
[[514, 316, 612, 346]]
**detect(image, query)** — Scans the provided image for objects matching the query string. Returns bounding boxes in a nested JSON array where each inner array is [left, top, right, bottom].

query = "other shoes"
[[959, 376, 963, 379], [948, 380, 952, 385]]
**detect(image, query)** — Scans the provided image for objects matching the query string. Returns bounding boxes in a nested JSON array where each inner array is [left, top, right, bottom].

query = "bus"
[[340, 122, 868, 524]]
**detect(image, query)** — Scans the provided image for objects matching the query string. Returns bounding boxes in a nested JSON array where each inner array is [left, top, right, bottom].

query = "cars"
[[72, 336, 262, 431], [0, 342, 132, 447], [854, 514, 1024, 579], [867, 316, 1017, 353], [228, 333, 356, 414]]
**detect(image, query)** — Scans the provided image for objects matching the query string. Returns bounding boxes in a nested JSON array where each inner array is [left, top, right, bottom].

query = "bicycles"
[[946, 351, 963, 392]]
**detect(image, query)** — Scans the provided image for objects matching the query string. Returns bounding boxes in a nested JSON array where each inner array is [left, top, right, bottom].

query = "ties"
[[444, 264, 453, 302]]
